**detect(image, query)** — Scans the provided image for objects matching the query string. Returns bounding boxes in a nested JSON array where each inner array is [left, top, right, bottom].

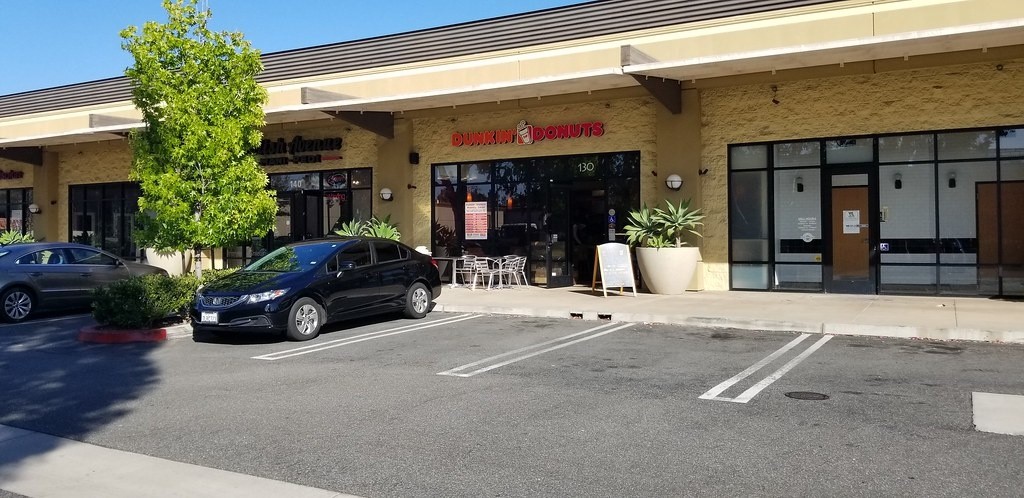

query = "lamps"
[[379, 188, 393, 201], [665, 174, 683, 191], [29, 204, 40, 214]]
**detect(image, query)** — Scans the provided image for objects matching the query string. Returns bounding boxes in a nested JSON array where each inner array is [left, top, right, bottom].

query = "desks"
[[433, 257, 464, 288], [488, 257, 514, 288]]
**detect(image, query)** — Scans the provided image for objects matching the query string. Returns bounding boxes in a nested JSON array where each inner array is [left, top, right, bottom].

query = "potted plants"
[[623, 197, 706, 295]]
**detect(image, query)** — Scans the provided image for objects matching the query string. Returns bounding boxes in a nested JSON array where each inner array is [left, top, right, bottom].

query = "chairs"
[[456, 255, 528, 290], [48, 252, 60, 264]]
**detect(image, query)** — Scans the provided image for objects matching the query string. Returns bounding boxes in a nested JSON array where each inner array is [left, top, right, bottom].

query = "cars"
[[189, 235, 443, 340], [0, 242, 175, 323], [499, 223, 538, 239]]
